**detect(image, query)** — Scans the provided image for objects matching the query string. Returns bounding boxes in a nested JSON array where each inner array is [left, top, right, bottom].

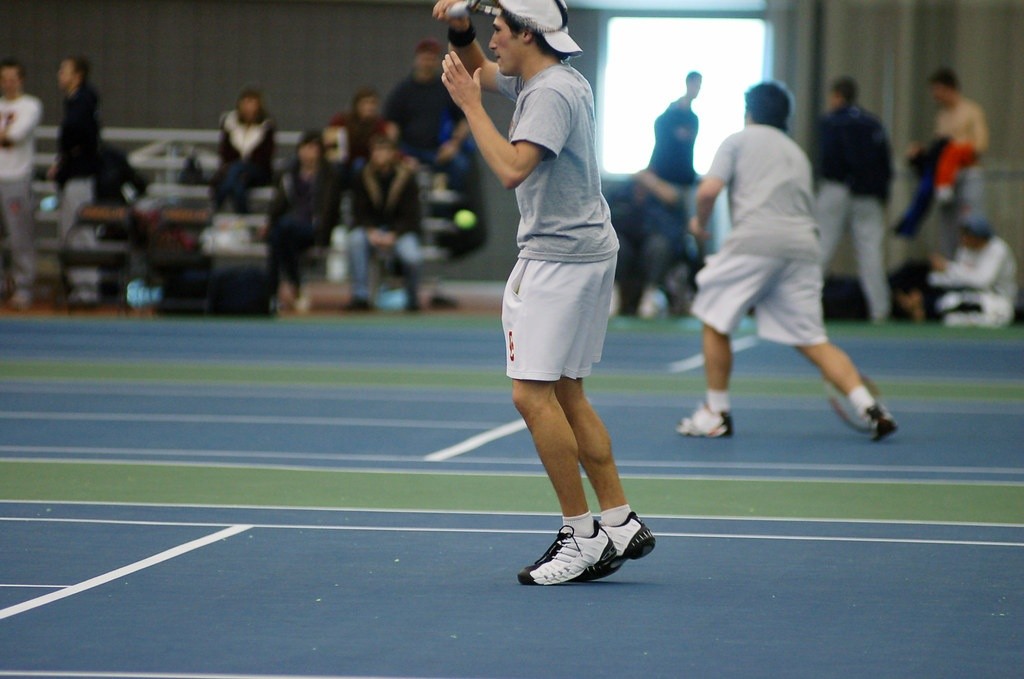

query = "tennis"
[[454, 210, 475, 228]]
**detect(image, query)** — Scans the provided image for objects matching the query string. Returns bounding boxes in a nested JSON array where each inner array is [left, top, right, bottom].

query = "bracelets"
[[446, 20, 477, 47]]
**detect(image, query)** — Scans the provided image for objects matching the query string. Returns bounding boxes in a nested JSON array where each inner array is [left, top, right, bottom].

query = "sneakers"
[[857, 405, 897, 440], [677, 408, 735, 439], [517, 520, 617, 586], [568, 511, 656, 582]]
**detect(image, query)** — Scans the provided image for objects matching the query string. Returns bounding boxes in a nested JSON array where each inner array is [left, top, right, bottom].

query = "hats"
[[497, 0, 584, 57]]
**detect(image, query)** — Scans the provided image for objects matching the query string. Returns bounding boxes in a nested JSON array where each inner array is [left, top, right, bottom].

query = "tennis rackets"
[[448, 0, 569, 34], [826, 375, 882, 431]]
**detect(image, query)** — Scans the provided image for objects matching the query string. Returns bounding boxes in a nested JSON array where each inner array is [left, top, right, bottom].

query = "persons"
[[688, 80, 905, 445], [890, 66, 990, 237], [790, 72, 901, 332], [599, 73, 708, 328], [426, 0, 656, 588], [0, 38, 483, 317], [892, 208, 1022, 330]]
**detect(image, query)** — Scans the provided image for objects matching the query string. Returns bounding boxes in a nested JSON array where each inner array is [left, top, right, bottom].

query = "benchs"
[[25, 123, 465, 312]]
